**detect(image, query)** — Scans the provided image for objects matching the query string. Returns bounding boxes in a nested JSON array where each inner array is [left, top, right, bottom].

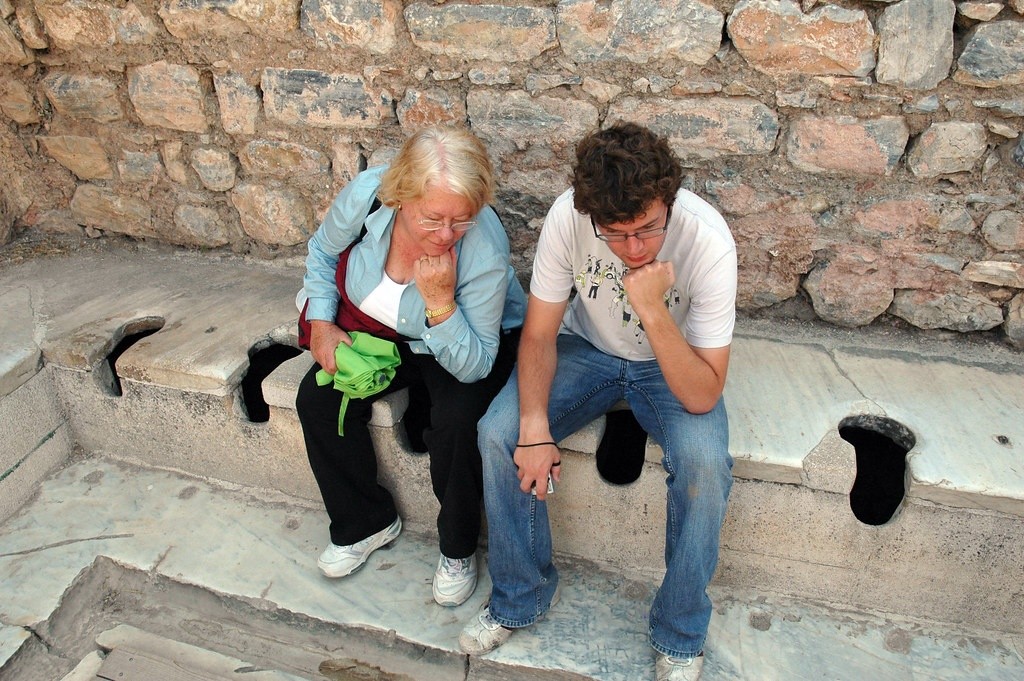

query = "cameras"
[[531, 472, 554, 495]]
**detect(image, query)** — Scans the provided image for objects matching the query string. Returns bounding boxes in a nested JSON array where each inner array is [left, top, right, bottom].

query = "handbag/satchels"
[[298, 190, 403, 354]]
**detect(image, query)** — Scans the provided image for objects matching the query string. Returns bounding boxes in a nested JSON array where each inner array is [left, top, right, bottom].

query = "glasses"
[[590, 205, 671, 242], [412, 199, 476, 231]]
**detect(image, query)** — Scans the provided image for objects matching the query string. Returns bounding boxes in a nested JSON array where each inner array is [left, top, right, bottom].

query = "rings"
[[420, 258, 428, 263]]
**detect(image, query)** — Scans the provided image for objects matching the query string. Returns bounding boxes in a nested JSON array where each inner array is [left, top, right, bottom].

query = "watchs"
[[424, 302, 456, 319]]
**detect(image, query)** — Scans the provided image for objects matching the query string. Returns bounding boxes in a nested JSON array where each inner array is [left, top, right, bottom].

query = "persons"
[[295, 124, 531, 606], [457, 121, 738, 681]]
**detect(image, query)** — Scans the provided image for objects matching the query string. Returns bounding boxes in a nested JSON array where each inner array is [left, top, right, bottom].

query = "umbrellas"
[[316, 331, 402, 436]]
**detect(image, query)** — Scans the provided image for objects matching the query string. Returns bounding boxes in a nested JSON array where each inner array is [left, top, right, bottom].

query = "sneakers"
[[458, 585, 561, 656], [432, 547, 478, 607], [655, 636, 702, 681], [316, 512, 402, 578]]
